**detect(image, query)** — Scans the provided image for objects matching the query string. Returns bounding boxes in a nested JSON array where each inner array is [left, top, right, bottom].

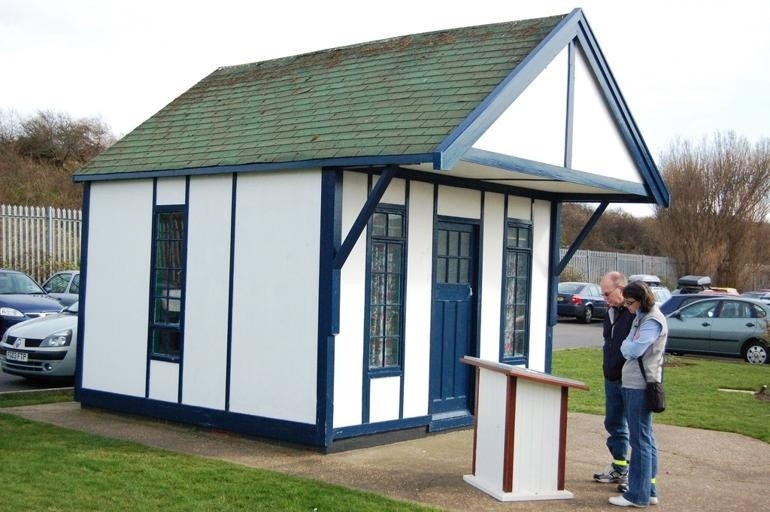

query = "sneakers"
[[593, 463, 628, 484], [608, 484, 658, 508]]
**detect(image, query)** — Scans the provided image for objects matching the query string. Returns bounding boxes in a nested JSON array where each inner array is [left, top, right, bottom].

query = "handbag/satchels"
[[646, 381, 665, 413]]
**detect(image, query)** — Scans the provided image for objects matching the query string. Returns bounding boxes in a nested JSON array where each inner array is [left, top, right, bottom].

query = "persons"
[[609, 279, 668, 508], [594, 272, 638, 493]]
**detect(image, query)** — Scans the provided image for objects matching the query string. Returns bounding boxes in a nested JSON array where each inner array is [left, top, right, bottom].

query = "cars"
[[40, 269, 80, 309], [0, 299, 79, 387], [551, 271, 770, 366], [0, 268, 66, 346]]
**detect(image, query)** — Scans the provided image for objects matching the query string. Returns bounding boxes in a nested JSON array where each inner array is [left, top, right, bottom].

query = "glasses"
[[624, 299, 639, 307], [601, 287, 618, 297]]
